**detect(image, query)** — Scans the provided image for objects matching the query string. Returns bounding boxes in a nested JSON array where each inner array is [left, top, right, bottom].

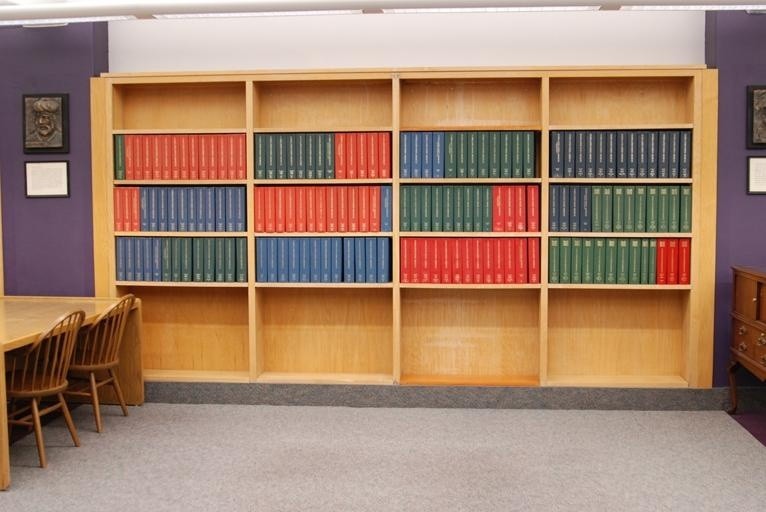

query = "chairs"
[[5, 309, 85, 469], [49, 293, 136, 433]]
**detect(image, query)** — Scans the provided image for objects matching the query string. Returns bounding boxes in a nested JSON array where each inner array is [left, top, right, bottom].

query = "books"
[[255, 133, 392, 282], [399, 132, 540, 283], [549, 130, 692, 283], [114, 135, 246, 282]]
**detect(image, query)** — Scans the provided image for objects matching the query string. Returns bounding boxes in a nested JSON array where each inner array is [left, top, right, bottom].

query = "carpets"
[[0, 402, 766, 512]]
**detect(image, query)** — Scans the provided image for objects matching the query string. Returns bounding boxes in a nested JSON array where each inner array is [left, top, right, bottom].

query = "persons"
[[26, 100, 63, 146]]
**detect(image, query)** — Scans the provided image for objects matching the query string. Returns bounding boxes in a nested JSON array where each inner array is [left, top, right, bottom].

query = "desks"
[[0, 295, 145, 491]]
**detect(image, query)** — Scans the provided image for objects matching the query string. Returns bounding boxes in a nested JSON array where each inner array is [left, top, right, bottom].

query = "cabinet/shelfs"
[[90, 64, 719, 390], [727, 263, 766, 414]]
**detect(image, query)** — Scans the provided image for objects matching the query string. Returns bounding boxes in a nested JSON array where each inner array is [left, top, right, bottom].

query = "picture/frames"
[[745, 85, 766, 149], [22, 93, 70, 155], [746, 156, 766, 195], [24, 160, 70, 198]]
